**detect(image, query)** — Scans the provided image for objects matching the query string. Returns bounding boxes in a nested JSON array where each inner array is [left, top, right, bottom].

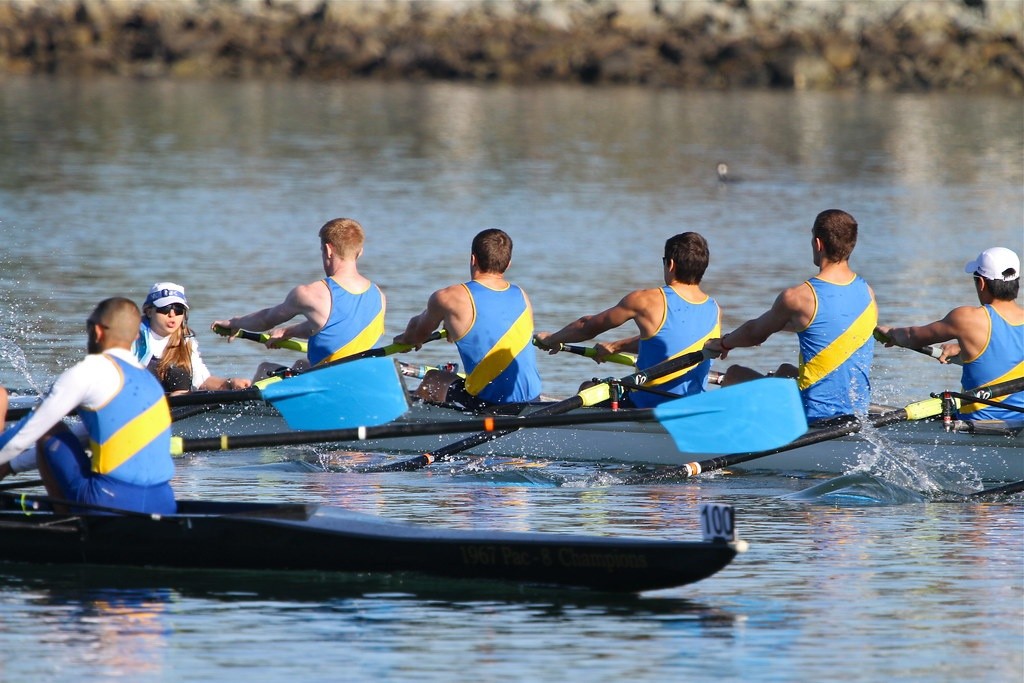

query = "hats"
[[964, 247, 1021, 282], [146, 283, 189, 313]]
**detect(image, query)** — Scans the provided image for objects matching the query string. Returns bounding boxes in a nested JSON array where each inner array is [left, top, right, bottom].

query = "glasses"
[[155, 303, 186, 316]]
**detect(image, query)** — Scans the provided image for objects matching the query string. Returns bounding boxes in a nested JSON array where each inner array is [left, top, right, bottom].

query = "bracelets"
[[227, 377, 233, 392], [720, 334, 734, 351]]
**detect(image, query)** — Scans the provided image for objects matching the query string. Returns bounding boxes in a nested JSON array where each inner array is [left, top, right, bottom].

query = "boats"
[[0, 492, 750, 601], [4, 385, 1023, 503]]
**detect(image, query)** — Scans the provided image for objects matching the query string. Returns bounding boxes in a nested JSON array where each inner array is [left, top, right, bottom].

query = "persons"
[[0, 298, 176, 519], [211, 217, 386, 385], [532, 231, 722, 408], [703, 209, 878, 429], [872, 247, 1024, 419], [393, 228, 542, 414], [132, 281, 251, 396]]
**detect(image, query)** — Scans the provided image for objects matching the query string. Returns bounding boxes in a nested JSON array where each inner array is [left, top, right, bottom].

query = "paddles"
[[0, 325, 1024, 514]]
